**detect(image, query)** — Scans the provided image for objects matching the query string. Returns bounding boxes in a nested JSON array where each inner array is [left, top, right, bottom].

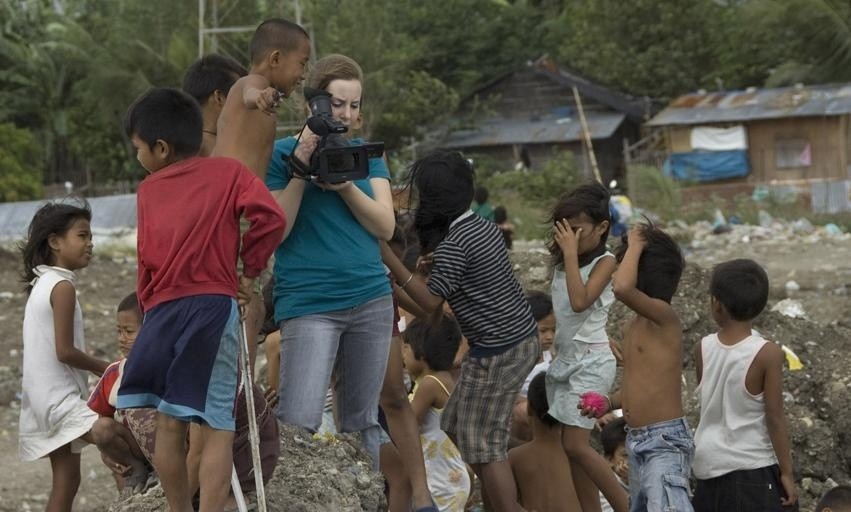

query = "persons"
[[265, 53, 396, 472], [214, 18, 312, 185], [18, 150, 850, 511], [182, 53, 248, 157], [117, 88, 285, 510]]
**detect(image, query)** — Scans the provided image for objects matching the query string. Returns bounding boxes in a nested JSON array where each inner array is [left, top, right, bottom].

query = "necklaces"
[[203, 130, 217, 136]]
